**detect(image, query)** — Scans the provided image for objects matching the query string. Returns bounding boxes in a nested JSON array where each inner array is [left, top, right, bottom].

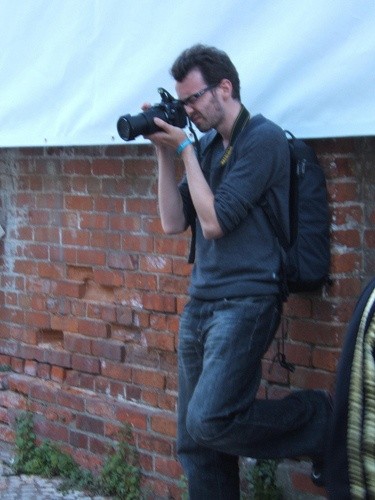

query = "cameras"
[[115, 87, 187, 141]]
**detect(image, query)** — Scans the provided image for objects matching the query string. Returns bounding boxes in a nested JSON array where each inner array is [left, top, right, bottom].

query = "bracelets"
[[176, 139, 191, 154]]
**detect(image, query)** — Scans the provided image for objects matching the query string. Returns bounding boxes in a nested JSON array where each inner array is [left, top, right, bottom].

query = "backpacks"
[[226, 119, 331, 293]]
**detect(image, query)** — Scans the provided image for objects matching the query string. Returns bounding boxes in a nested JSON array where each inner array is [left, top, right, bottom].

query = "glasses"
[[183, 83, 219, 107]]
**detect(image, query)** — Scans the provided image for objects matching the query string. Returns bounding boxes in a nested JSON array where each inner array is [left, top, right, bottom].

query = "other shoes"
[[311, 391, 335, 487]]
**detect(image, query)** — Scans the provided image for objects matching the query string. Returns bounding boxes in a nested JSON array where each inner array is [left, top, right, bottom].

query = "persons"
[[140, 45, 333, 500]]
